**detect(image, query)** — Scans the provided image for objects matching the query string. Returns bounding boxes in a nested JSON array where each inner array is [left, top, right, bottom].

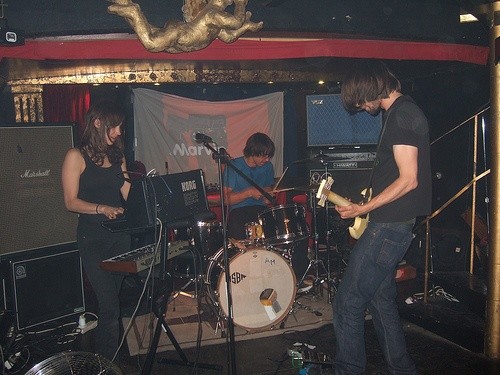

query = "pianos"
[[99, 212, 225, 375]]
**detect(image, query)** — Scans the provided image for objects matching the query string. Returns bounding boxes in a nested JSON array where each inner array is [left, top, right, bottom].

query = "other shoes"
[[296, 281, 313, 293]]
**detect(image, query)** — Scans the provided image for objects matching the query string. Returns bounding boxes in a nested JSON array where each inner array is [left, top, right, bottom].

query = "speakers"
[[303, 91, 386, 149], [1, 240, 84, 336], [310, 158, 373, 210], [1, 123, 81, 259]]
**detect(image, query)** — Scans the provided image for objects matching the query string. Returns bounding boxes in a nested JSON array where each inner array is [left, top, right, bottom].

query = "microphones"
[[115, 171, 147, 178], [193, 131, 212, 144], [165, 162, 168, 175], [4, 351, 23, 370]]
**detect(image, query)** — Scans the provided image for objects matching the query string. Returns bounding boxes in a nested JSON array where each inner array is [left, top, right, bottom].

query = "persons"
[[220, 132, 278, 252], [333, 59, 432, 375], [62, 99, 132, 366]]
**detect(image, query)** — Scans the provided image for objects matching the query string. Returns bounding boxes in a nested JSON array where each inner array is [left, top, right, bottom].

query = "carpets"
[[122, 291, 372, 358]]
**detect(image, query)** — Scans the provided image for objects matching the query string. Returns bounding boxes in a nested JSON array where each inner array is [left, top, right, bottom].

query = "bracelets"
[[96, 203, 101, 215]]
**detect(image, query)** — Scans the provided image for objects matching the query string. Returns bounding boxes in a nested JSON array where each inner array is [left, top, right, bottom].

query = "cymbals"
[[294, 184, 320, 191]]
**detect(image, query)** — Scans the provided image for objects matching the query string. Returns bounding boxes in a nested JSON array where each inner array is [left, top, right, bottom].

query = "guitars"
[[316, 179, 372, 240]]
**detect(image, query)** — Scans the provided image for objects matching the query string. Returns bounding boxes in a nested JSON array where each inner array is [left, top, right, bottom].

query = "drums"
[[259, 202, 311, 245], [168, 221, 224, 278], [205, 241, 298, 330], [244, 221, 265, 241], [313, 206, 354, 243]]
